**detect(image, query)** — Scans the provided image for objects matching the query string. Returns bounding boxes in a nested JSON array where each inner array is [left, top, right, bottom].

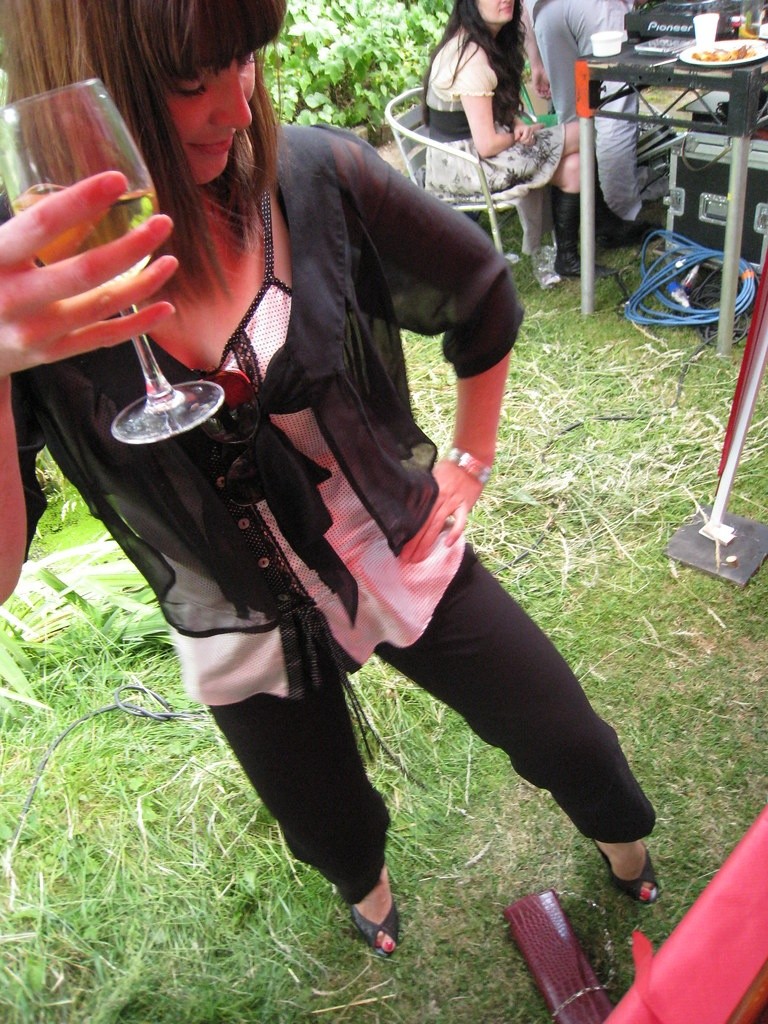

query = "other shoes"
[[592, 837, 657, 904], [352, 895, 399, 958]]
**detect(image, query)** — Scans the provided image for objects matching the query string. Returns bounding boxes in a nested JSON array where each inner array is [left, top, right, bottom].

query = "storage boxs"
[[661, 133, 768, 275]]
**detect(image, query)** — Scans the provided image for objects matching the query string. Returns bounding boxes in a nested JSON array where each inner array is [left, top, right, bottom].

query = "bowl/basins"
[[591, 30, 623, 57]]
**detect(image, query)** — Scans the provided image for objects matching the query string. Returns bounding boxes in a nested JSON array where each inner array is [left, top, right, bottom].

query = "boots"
[[551, 185, 619, 279], [593, 150, 665, 248]]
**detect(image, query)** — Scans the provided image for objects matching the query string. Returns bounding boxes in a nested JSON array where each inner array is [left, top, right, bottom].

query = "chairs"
[[384, 87, 558, 267]]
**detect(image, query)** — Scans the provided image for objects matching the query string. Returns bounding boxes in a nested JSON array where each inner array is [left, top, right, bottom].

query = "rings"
[[531, 135, 535, 139]]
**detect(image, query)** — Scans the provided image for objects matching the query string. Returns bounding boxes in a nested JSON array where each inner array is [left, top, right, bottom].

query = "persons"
[[421, 0, 667, 278], [0, 0, 661, 955]]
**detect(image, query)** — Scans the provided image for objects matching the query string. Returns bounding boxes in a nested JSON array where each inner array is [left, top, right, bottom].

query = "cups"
[[738, 1, 764, 39], [693, 13, 719, 54]]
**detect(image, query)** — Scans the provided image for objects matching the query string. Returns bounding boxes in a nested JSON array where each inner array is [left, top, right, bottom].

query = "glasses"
[[191, 368, 277, 507]]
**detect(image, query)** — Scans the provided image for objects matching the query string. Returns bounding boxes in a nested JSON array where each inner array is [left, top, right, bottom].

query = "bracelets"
[[443, 449, 492, 488]]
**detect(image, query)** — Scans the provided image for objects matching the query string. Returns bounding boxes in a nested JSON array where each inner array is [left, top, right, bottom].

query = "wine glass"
[[0, 78, 226, 446]]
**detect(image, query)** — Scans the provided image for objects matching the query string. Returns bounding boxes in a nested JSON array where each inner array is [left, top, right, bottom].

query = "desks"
[[573, 36, 768, 358]]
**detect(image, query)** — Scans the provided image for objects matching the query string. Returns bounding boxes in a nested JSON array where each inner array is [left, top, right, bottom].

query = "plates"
[[679, 39, 768, 67]]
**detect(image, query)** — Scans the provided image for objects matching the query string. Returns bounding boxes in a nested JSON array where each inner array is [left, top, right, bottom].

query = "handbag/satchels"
[[503, 888, 618, 1024]]
